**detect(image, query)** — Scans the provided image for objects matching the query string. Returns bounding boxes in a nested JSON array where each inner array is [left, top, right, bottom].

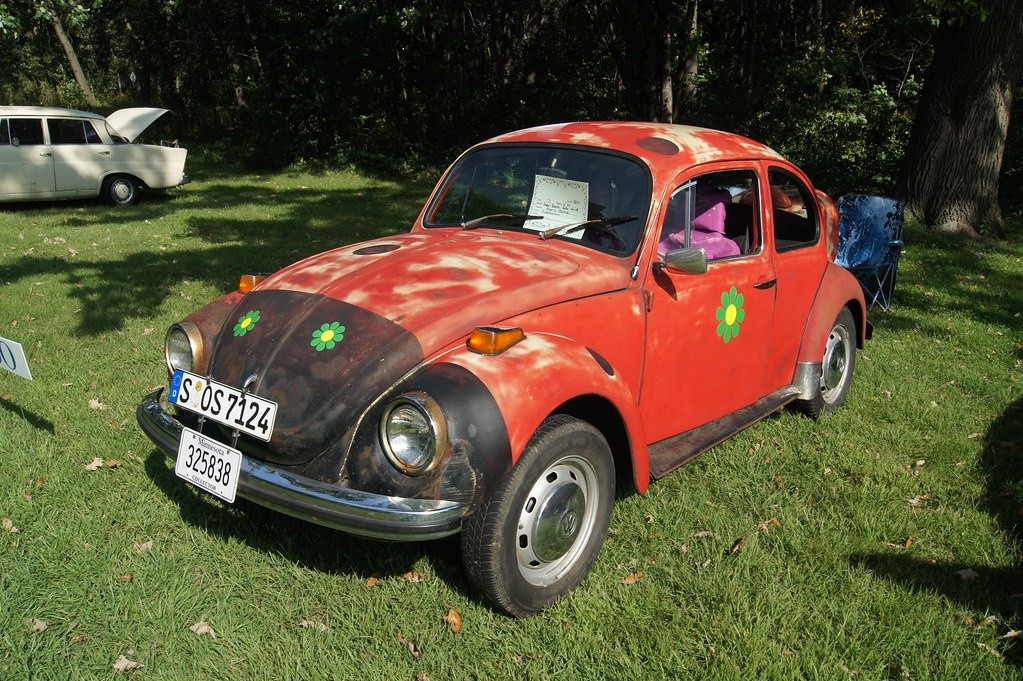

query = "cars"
[[0, 105, 189, 206], [133, 119, 870, 617]]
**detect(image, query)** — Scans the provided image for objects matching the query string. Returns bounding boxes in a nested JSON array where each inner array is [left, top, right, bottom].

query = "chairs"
[[655, 186, 743, 263], [831, 191, 903, 313]]
